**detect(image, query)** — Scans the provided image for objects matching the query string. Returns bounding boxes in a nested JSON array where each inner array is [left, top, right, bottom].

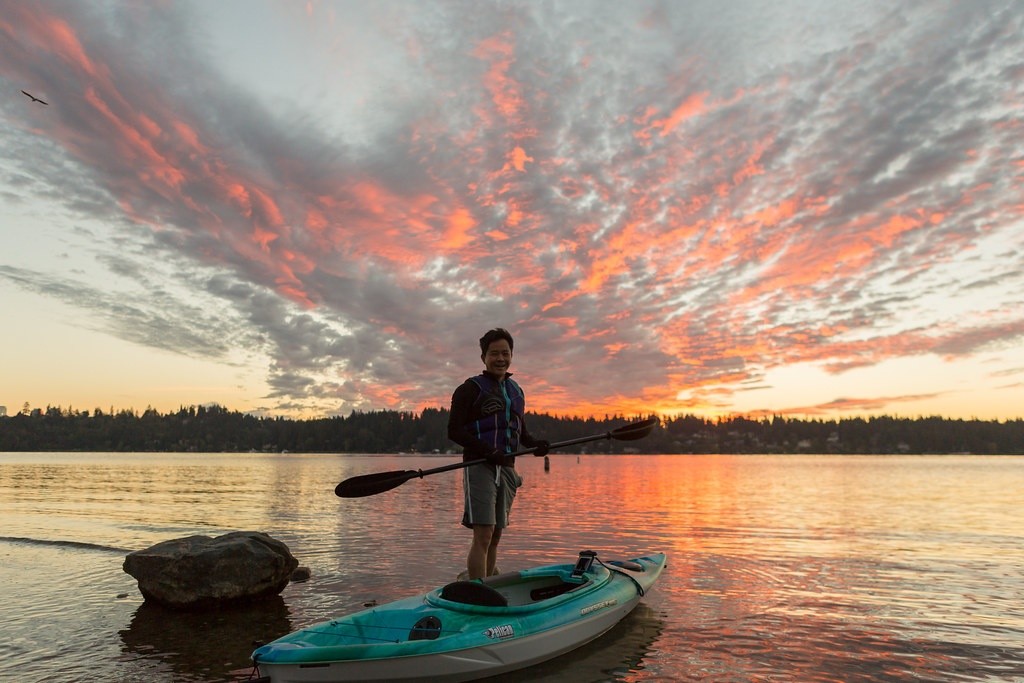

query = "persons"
[[445, 327, 553, 577]]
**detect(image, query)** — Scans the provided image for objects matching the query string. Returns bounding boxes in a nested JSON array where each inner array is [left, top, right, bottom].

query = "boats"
[[248, 550, 668, 683]]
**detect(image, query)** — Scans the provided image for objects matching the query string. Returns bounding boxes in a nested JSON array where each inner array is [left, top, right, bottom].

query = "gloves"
[[528, 439, 549, 457], [487, 449, 509, 465]]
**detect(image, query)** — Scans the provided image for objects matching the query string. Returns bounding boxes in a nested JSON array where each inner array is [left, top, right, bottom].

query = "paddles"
[[333, 418, 658, 499]]
